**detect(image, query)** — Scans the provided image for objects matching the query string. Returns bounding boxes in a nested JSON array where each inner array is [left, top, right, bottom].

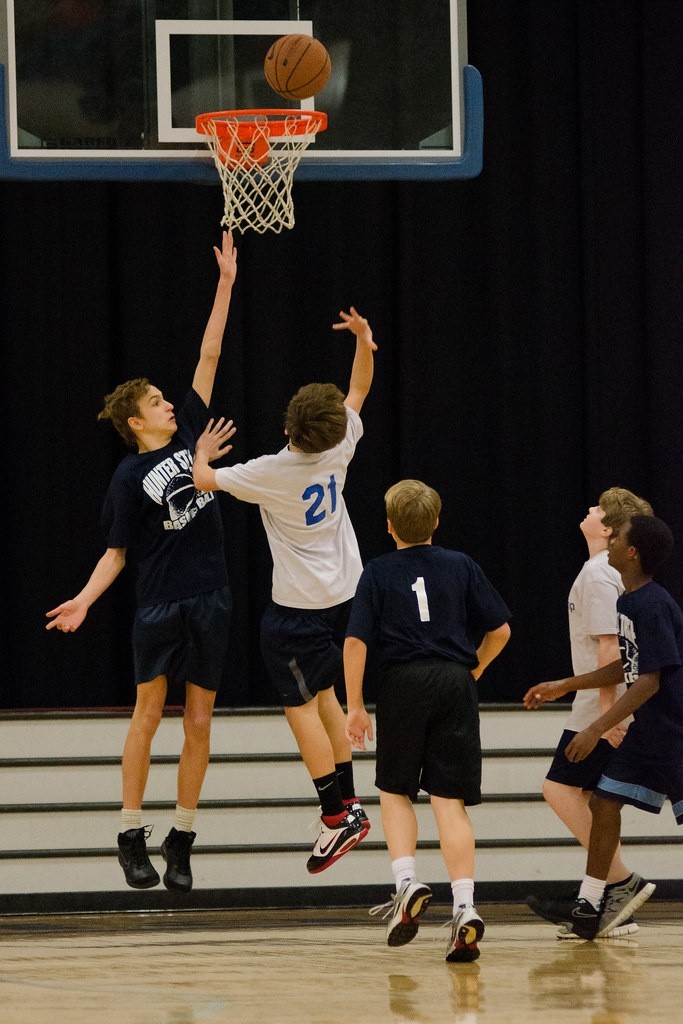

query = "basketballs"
[[263, 33, 332, 102]]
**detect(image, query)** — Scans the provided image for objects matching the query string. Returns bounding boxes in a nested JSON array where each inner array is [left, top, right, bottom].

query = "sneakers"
[[369, 879, 432, 947], [596, 871, 657, 938], [435, 905, 485, 962], [557, 906, 639, 938], [160, 827, 196, 894], [528, 893, 608, 941], [118, 825, 160, 889], [306, 798, 371, 874]]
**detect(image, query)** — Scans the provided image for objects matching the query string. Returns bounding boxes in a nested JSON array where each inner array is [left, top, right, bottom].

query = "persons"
[[345, 479, 513, 962], [521, 488, 656, 938], [192, 308, 379, 873], [523, 516, 683, 940], [44, 228, 238, 896]]
